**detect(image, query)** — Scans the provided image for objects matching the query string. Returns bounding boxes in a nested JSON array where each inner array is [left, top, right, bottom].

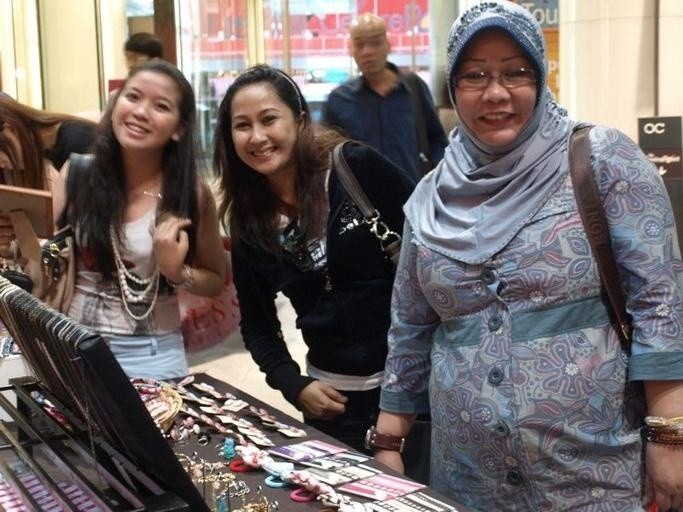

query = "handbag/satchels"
[[41, 224, 74, 284]]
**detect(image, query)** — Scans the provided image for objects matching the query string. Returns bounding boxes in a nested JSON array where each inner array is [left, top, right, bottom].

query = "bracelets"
[[641, 416, 683, 444]]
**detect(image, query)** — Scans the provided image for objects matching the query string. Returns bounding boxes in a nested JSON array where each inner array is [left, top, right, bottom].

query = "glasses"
[[456, 64, 535, 91]]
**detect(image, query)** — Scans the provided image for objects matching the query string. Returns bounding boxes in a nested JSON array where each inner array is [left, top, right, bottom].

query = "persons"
[[373, 0, 683, 512]]
